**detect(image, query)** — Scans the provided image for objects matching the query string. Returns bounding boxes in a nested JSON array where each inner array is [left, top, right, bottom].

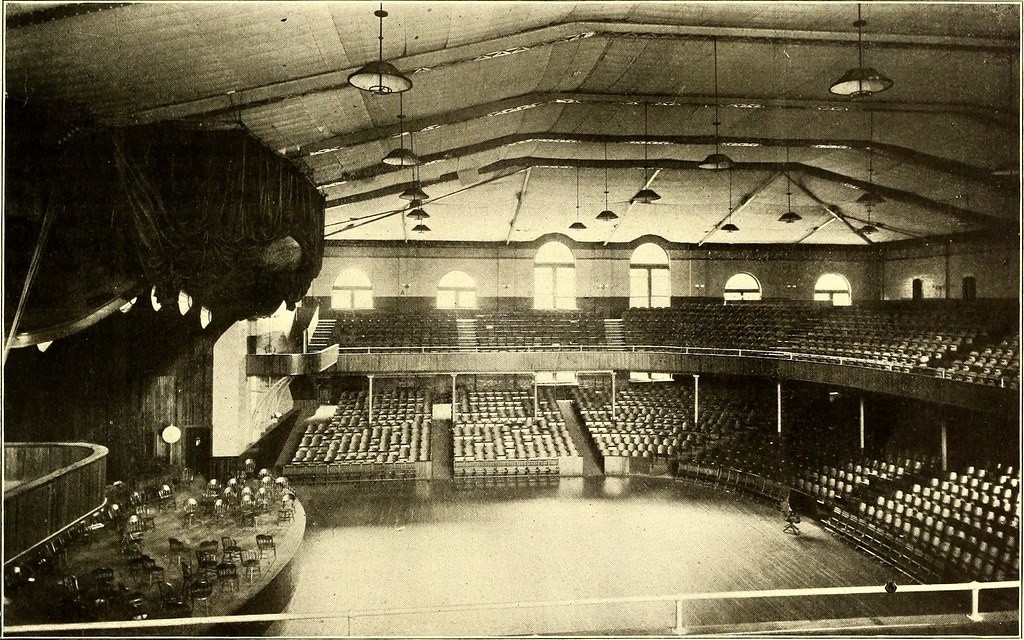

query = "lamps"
[[412, 218, 431, 231], [399, 131, 429, 202], [721, 170, 740, 233], [828, 4, 894, 98], [407, 166, 430, 220], [596, 143, 618, 222], [777, 147, 802, 224], [859, 206, 879, 235], [569, 168, 587, 230], [347, 1, 414, 95], [381, 94, 423, 168], [855, 110, 887, 206], [697, 40, 736, 173], [631, 104, 661, 204]]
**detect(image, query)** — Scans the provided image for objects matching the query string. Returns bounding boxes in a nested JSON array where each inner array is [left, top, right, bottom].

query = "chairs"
[[6, 458, 262, 636], [255, 534, 277, 561], [260, 476, 275, 492], [258, 468, 272, 480], [290, 310, 1019, 582], [282, 493, 297, 516], [273, 477, 289, 504], [256, 488, 272, 516]]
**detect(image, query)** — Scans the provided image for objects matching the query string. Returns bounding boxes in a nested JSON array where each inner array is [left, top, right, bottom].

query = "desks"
[[276, 508, 291, 526]]
